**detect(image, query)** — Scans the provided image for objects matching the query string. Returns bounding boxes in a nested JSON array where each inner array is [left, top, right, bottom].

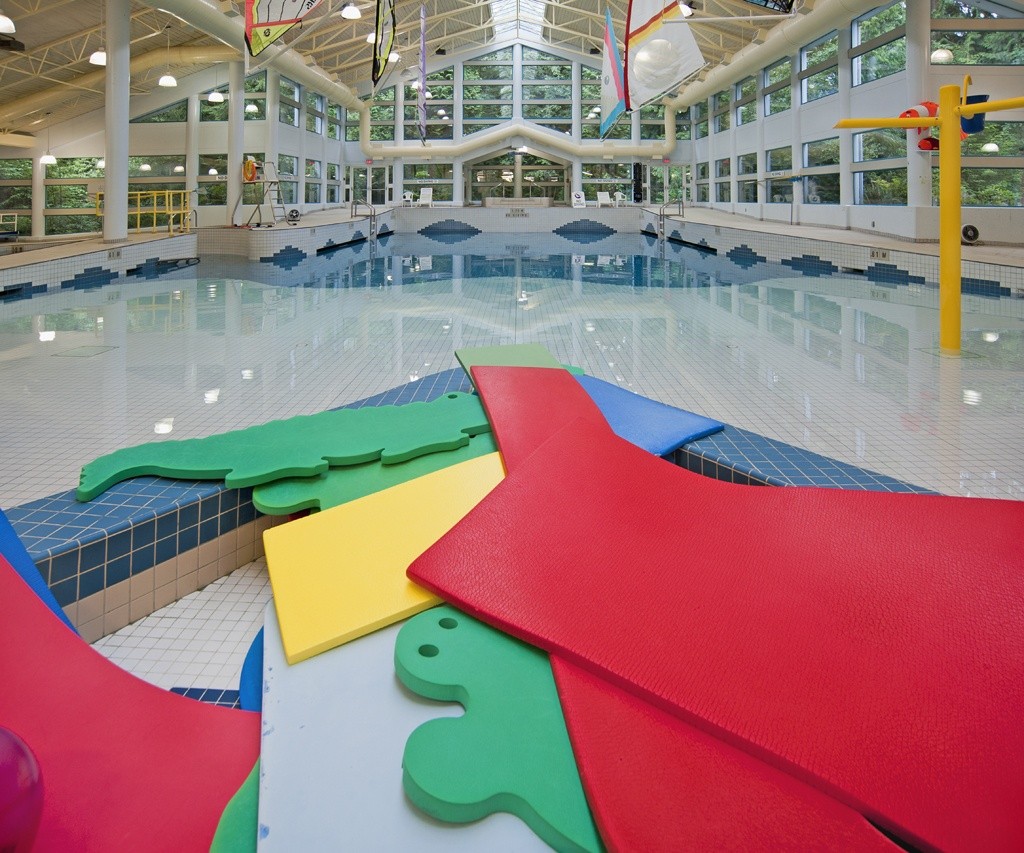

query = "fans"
[[960, 223, 980, 245]]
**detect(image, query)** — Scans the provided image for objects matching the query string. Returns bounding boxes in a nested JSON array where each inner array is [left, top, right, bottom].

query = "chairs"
[[773, 194, 785, 203], [808, 195, 821, 204], [402, 191, 414, 208], [415, 187, 435, 208], [784, 193, 793, 203], [614, 191, 627, 207], [595, 191, 614, 209]]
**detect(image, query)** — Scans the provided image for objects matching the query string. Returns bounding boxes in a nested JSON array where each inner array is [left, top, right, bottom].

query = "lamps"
[[678, 3, 693, 17], [366, 25, 384, 44], [244, 99, 259, 112], [89, 0, 107, 66], [425, 90, 434, 99], [0, 7, 16, 34], [158, 25, 178, 87], [387, 42, 403, 63], [586, 106, 601, 119], [39, 112, 58, 165], [208, 168, 219, 176], [208, 62, 225, 102], [982, 138, 1000, 153], [341, 0, 361, 20], [172, 165, 185, 173], [411, 79, 419, 89], [96, 159, 105, 169], [137, 162, 152, 171], [437, 107, 453, 126], [400, 38, 414, 78]]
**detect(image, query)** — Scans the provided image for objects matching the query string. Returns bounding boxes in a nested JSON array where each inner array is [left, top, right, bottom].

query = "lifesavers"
[[244, 161, 256, 182]]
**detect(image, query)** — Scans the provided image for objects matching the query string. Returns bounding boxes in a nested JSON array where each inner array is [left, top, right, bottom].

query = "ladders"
[[232, 161, 291, 228]]
